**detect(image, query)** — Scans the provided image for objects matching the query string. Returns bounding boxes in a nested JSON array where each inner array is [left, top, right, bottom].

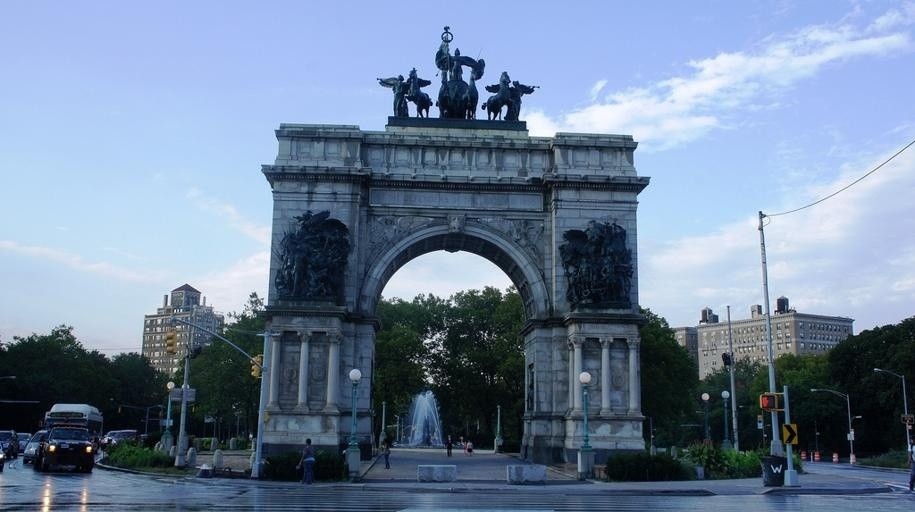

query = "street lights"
[[872, 368, 913, 463], [809, 388, 863, 465], [0, 375, 18, 381], [719, 390, 733, 448], [493, 405, 506, 454], [576, 370, 597, 480], [701, 391, 713, 442], [393, 415, 400, 442], [159, 380, 176, 451], [378, 401, 394, 449], [344, 366, 363, 479], [145, 404, 166, 433]]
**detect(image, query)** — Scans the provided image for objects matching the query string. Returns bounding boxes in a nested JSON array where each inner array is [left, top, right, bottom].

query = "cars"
[[0, 428, 149, 474]]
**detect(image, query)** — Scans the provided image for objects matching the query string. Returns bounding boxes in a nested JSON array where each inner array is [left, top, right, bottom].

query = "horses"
[[402, 70, 433, 118], [481, 71, 516, 120], [463, 72, 478, 119], [438, 70, 453, 117]]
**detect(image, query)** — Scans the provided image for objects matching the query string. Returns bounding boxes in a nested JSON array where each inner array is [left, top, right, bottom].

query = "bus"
[[39, 402, 104, 442]]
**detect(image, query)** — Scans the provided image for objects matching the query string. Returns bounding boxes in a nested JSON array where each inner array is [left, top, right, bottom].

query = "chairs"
[[72, 431, 82, 439]]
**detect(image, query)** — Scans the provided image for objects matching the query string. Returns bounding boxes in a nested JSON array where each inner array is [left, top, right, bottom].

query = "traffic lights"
[[249, 355, 262, 377], [191, 345, 202, 359], [165, 328, 178, 355], [758, 393, 779, 410]]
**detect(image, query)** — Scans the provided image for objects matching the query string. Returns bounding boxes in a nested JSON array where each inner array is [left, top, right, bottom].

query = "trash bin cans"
[[760, 456, 787, 486]]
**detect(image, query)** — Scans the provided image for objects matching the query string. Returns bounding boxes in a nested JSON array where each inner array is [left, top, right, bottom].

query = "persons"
[[380, 74, 409, 117], [452, 48, 463, 80], [445, 437, 452, 456], [296, 439, 315, 485], [908, 436, 915, 490], [504, 81, 522, 121], [248, 432, 256, 469], [464, 440, 473, 456], [384, 438, 390, 469]]
